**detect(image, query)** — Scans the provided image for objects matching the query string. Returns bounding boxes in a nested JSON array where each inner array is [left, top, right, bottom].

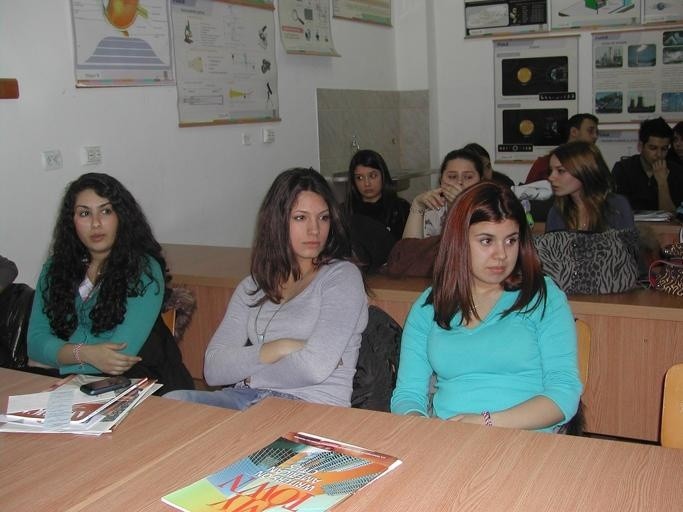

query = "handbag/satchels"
[[386, 235, 442, 278], [648, 243, 683, 297], [528, 227, 642, 296]]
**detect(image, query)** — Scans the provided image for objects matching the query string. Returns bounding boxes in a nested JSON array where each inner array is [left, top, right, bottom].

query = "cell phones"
[[80, 376, 131, 395]]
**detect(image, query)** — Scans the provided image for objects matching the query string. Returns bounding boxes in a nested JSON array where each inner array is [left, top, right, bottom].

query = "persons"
[[612, 118, 683, 212], [337, 150, 412, 267], [19, 174, 196, 396], [163, 167, 376, 411], [546, 141, 646, 294], [464, 143, 514, 187], [525, 114, 598, 222], [667, 121, 683, 166], [386, 150, 486, 279], [390, 178, 584, 434]]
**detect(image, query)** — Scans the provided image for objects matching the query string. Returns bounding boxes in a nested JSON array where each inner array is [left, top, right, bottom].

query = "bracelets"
[[72, 342, 83, 364], [481, 410, 492, 427], [410, 207, 426, 216]]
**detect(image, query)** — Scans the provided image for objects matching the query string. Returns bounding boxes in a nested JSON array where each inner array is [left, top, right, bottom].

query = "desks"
[[76, 393, 681, 512], [0, 364, 242, 512], [522, 216, 680, 253], [145, 243, 683, 445]]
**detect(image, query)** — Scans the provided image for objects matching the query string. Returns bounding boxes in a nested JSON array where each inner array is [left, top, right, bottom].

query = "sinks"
[[332, 170, 409, 191]]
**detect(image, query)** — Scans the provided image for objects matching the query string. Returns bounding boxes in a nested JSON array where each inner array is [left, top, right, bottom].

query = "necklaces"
[[578, 223, 586, 231], [254, 281, 312, 345], [469, 304, 482, 313]]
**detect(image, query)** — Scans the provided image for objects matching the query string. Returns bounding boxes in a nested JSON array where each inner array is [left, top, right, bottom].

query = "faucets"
[[351, 136, 361, 150]]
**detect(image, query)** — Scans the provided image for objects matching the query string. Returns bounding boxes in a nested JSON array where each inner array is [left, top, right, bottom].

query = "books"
[[161, 431, 402, 512], [0, 374, 165, 436]]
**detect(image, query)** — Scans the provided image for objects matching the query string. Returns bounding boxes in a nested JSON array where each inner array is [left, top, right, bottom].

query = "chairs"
[[570, 318, 594, 394], [654, 362, 683, 446], [161, 301, 178, 334]]
